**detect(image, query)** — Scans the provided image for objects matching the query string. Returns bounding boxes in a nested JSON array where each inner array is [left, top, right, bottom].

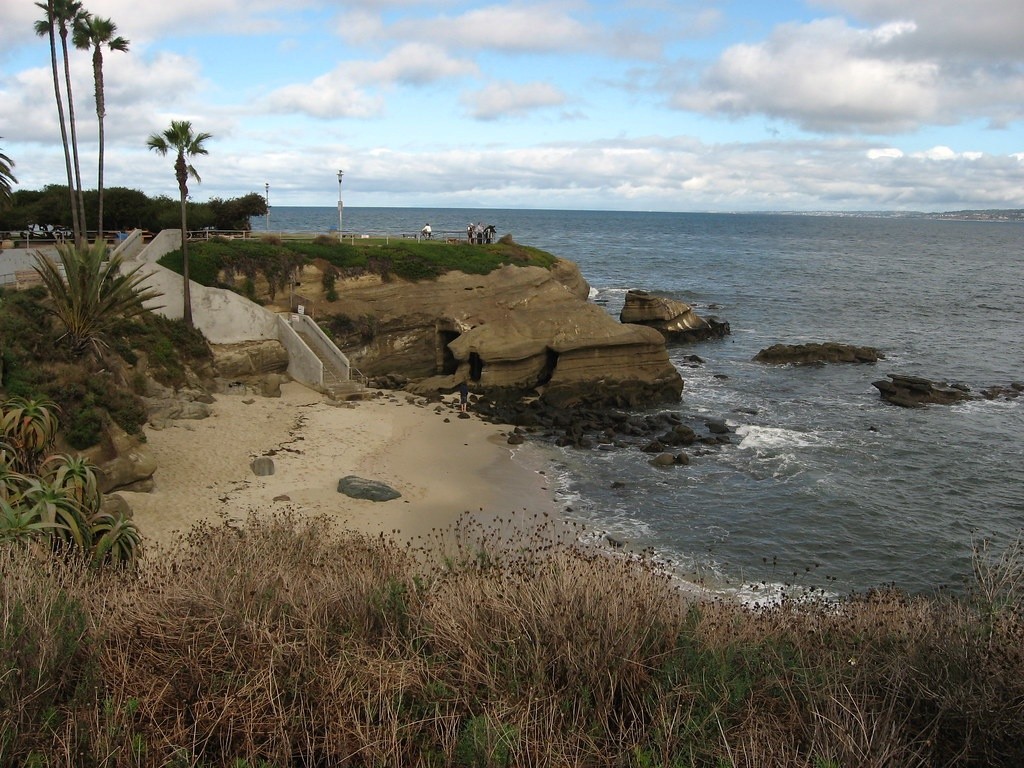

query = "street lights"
[[336, 170, 344, 242], [264, 182, 270, 229]]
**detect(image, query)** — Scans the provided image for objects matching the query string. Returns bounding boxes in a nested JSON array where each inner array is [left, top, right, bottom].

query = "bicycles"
[[420, 232, 435, 241]]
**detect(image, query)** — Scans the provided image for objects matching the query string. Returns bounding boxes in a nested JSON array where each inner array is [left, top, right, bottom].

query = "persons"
[[467, 222, 497, 244], [422, 223, 432, 238], [459, 381, 468, 412]]
